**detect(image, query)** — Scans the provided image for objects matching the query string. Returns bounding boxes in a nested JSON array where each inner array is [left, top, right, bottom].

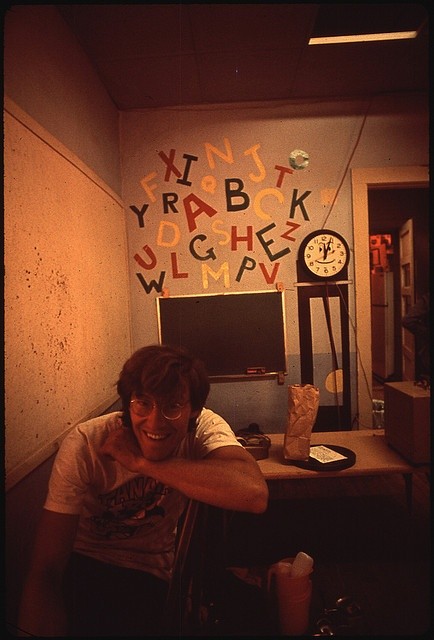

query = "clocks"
[[297, 229, 351, 281]]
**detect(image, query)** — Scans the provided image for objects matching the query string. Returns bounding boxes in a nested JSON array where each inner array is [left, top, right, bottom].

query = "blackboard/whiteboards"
[[157, 289, 289, 381]]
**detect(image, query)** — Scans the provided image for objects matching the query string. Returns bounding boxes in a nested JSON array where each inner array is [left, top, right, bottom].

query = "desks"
[[219, 428, 410, 546]]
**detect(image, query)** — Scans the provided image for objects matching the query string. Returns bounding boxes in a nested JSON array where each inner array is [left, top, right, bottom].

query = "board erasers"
[[246, 367, 267, 373]]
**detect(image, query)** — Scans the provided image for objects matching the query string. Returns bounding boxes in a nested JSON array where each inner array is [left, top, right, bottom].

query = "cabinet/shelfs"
[[382, 378, 430, 465]]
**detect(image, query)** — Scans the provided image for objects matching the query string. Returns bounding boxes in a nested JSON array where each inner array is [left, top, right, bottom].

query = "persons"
[[17, 343, 271, 639]]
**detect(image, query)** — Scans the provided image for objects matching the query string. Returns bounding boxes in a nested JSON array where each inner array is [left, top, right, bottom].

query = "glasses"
[[128, 399, 191, 420]]
